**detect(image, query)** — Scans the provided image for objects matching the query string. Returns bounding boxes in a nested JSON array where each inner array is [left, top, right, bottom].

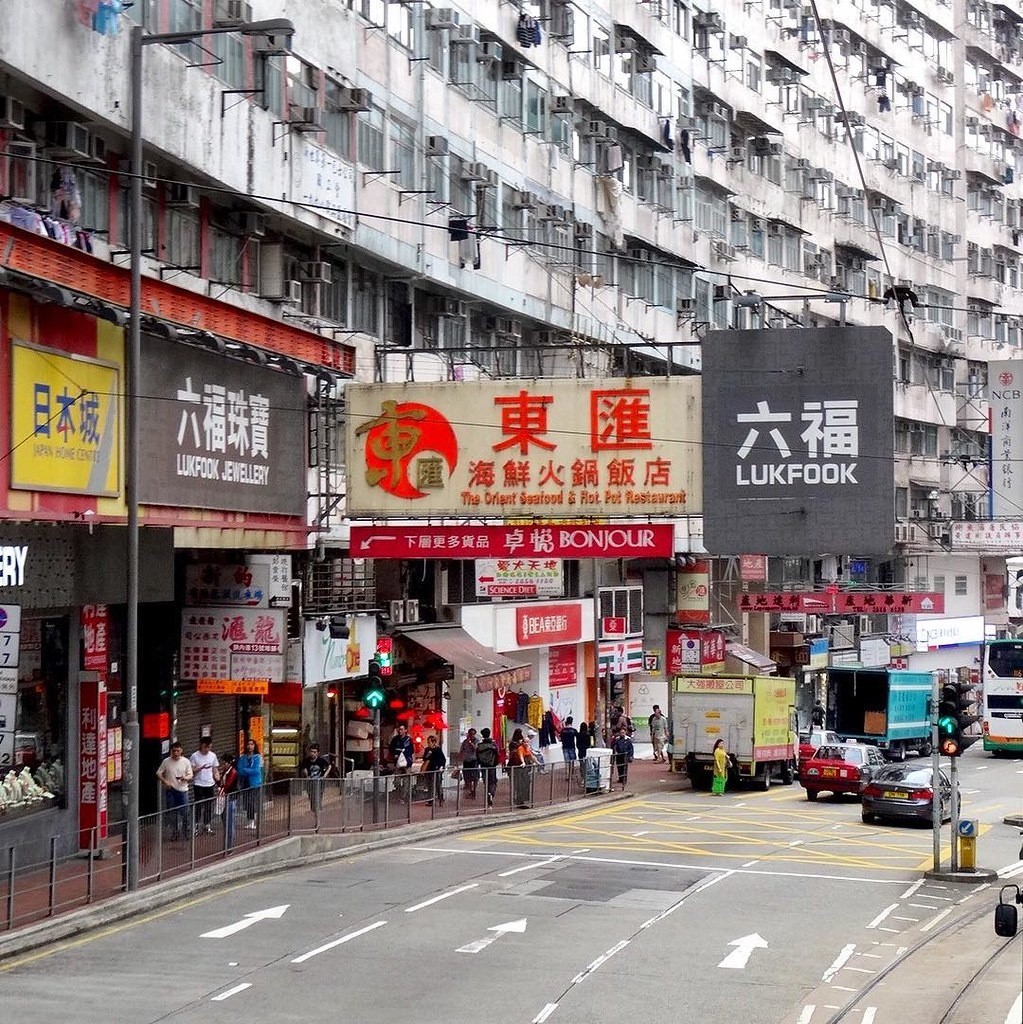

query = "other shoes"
[[245, 823, 256, 830], [517, 806, 529, 809], [440, 800, 443, 807], [184, 830, 191, 839], [170, 833, 180, 841], [488, 792, 493, 805], [426, 802, 433, 805], [204, 825, 212, 833]]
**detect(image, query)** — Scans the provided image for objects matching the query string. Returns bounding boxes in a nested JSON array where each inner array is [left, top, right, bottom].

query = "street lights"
[[119, 17, 297, 891]]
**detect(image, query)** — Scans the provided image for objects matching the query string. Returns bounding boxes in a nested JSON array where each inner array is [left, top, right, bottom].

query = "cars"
[[800, 729, 888, 803], [860, 765, 962, 827]]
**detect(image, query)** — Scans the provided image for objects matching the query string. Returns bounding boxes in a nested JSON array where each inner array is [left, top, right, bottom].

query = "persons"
[[712, 739, 731, 796], [389, 726, 414, 805], [649, 705, 670, 764], [218, 754, 239, 856], [421, 736, 446, 806], [189, 736, 220, 836], [615, 728, 633, 783], [455, 728, 479, 799], [576, 722, 591, 774], [977, 699, 984, 734], [507, 729, 541, 809], [303, 743, 332, 828], [559, 716, 578, 780], [155, 742, 186, 841], [612, 707, 627, 732], [478, 729, 498, 806], [236, 739, 262, 829], [811, 700, 825, 729]]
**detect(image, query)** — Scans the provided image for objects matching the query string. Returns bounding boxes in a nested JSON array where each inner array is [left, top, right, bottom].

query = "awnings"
[[396, 620, 533, 693], [726, 641, 777, 674]]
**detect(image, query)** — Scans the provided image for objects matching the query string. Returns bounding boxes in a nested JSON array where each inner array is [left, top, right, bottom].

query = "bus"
[[983, 639, 1023, 756]]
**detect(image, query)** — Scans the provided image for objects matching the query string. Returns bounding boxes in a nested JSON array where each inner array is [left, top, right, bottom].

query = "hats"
[[528, 730, 538, 736]]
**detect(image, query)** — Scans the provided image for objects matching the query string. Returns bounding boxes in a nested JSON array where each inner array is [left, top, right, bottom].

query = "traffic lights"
[[939, 681, 982, 758], [367, 659, 386, 711]]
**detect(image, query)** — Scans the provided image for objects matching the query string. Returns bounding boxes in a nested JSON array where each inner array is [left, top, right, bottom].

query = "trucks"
[[822, 666, 936, 762], [669, 674, 800, 791]]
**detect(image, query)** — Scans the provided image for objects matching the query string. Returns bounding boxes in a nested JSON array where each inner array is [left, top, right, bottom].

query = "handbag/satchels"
[[397, 751, 407, 768], [452, 766, 462, 780], [214, 789, 225, 814], [420, 759, 430, 775]]
